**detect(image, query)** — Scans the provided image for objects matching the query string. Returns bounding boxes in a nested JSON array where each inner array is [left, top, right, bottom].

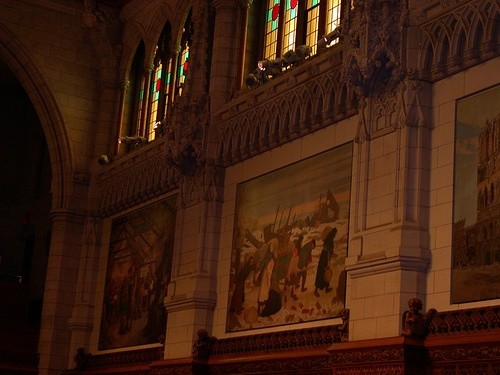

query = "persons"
[[401, 298, 431, 341], [192, 328, 215, 358], [337, 309, 349, 339], [75, 348, 88, 367]]
